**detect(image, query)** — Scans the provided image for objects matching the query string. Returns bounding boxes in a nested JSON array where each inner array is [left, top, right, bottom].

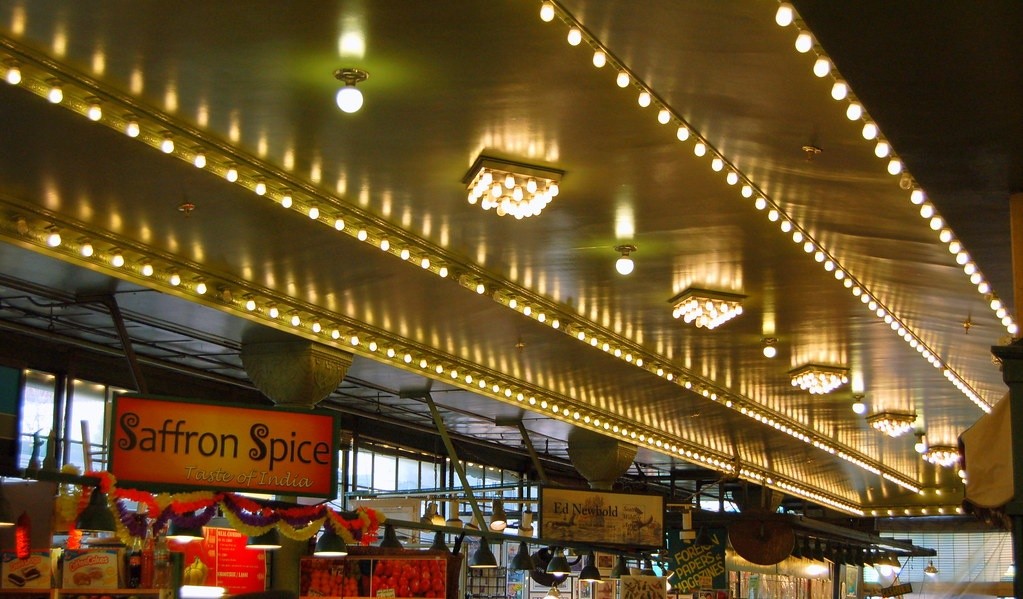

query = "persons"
[[167, 509, 203, 540], [75, 489, 115, 532]]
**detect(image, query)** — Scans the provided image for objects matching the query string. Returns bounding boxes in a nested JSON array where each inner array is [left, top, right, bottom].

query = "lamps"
[[334, 69, 369, 112], [762, 336, 961, 466], [70, 499, 940, 580], [614, 245, 636, 276], [461, 156, 564, 218], [669, 287, 749, 331]]
